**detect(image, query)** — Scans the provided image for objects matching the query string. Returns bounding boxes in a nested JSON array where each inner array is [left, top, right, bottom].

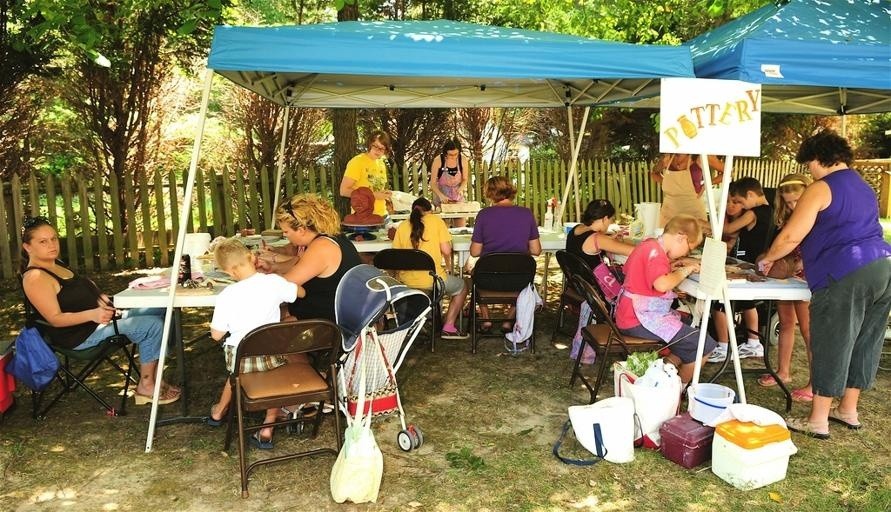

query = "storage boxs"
[[653, 409, 712, 470], [709, 418, 792, 492], [608, 350, 682, 439]]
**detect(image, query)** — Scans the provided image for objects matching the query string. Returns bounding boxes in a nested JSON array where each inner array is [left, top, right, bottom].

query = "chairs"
[[12, 276, 145, 422], [562, 271, 669, 411], [367, 246, 445, 355], [544, 243, 613, 355], [460, 250, 539, 355], [215, 313, 346, 501]]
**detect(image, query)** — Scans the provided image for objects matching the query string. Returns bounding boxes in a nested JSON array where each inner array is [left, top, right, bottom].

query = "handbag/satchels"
[[5, 324, 60, 392], [330, 423, 383, 505], [553, 397, 635, 466], [613, 361, 682, 450], [592, 262, 622, 300]]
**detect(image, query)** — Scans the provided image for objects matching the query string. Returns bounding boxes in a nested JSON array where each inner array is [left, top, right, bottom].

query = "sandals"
[[785, 416, 831, 439], [828, 407, 863, 429]]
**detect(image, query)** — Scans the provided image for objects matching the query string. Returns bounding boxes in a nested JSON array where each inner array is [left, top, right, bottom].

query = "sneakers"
[[441, 328, 470, 339], [738, 343, 766, 359], [707, 344, 733, 363]]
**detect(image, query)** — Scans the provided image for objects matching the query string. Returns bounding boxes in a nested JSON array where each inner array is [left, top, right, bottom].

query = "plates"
[[136, 278, 173, 289]]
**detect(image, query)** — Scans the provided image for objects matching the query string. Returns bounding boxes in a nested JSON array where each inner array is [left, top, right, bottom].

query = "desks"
[[562, 207, 813, 419], [376, 210, 490, 231], [110, 227, 292, 429], [344, 222, 613, 321]]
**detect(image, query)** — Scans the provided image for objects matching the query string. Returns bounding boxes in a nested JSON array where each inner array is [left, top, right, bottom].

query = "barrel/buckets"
[[182, 232, 212, 275], [686, 383, 736, 425]]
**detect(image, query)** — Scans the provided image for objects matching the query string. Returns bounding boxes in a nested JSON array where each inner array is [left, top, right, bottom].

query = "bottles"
[[545, 203, 554, 231]]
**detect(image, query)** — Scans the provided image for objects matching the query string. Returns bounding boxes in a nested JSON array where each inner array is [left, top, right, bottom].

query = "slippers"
[[785, 389, 813, 403], [758, 373, 792, 386], [207, 400, 225, 426], [134, 381, 181, 405], [247, 430, 274, 449]]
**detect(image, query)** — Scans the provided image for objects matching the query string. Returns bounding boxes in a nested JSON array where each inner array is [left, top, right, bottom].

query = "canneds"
[[178, 254, 191, 283]]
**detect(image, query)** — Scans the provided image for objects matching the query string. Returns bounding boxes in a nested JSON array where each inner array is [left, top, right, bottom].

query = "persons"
[[470, 174, 543, 336], [339, 130, 392, 219], [272, 189, 363, 423], [758, 133, 891, 441], [205, 238, 308, 449], [430, 140, 470, 230], [757, 171, 814, 405], [20, 218, 183, 407], [394, 197, 470, 341], [343, 186, 384, 226], [566, 198, 636, 305], [615, 152, 770, 404]]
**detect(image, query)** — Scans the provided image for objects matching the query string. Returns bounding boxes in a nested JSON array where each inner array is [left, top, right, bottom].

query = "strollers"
[[285, 258, 445, 452]]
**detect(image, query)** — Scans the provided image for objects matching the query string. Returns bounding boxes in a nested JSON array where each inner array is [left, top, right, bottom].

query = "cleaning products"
[[544, 198, 554, 231]]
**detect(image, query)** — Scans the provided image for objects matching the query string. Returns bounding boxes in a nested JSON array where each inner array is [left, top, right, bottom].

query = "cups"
[[637, 201, 662, 236]]
